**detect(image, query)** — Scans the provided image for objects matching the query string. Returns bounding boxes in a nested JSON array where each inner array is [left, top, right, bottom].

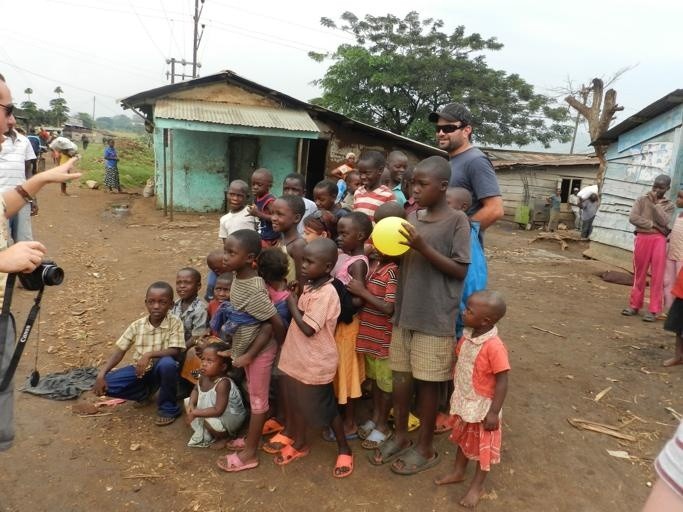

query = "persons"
[[661, 265, 682, 366], [656, 188, 683, 320], [547, 188, 561, 232], [433, 290, 510, 507], [429, 104, 504, 233], [0, 127, 89, 245], [104, 139, 123, 192], [577, 192, 599, 237], [172, 147, 487, 478], [640, 417, 683, 511], [0, 70, 80, 454], [92, 281, 186, 426], [568, 187, 582, 231], [621, 175, 675, 322]]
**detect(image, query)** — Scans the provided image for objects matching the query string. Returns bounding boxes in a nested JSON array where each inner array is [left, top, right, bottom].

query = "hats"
[[429, 103, 471, 124]]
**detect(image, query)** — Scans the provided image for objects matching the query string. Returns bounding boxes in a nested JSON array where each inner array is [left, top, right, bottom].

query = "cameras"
[[18, 261, 64, 291]]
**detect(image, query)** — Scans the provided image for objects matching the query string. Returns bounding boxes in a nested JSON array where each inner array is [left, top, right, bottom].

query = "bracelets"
[[13, 184, 32, 203]]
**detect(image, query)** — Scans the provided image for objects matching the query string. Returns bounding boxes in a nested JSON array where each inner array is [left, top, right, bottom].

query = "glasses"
[[0, 103, 12, 115], [435, 125, 462, 132]]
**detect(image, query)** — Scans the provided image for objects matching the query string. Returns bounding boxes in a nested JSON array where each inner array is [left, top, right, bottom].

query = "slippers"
[[332, 449, 353, 478], [322, 424, 357, 441], [643, 313, 660, 321], [154, 416, 175, 425], [357, 408, 453, 475], [216, 419, 310, 472], [132, 383, 159, 408], [621, 307, 638, 315]]
